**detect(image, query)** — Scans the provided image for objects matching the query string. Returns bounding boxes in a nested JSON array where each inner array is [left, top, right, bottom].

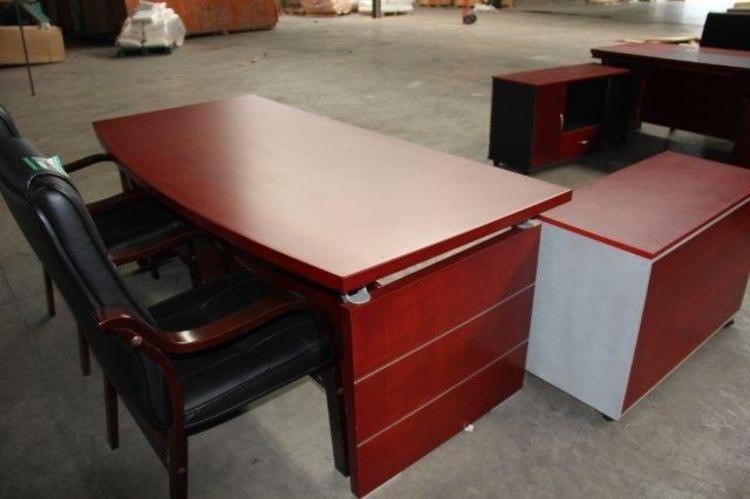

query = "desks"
[[90, 89, 573, 499], [589, 41, 747, 148]]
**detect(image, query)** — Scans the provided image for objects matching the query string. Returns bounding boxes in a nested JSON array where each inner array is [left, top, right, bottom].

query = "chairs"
[[2, 133, 348, 497], [3, 105, 211, 380]]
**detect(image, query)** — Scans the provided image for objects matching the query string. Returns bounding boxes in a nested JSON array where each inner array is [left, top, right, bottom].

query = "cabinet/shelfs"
[[522, 148, 748, 426], [488, 61, 634, 177]]
